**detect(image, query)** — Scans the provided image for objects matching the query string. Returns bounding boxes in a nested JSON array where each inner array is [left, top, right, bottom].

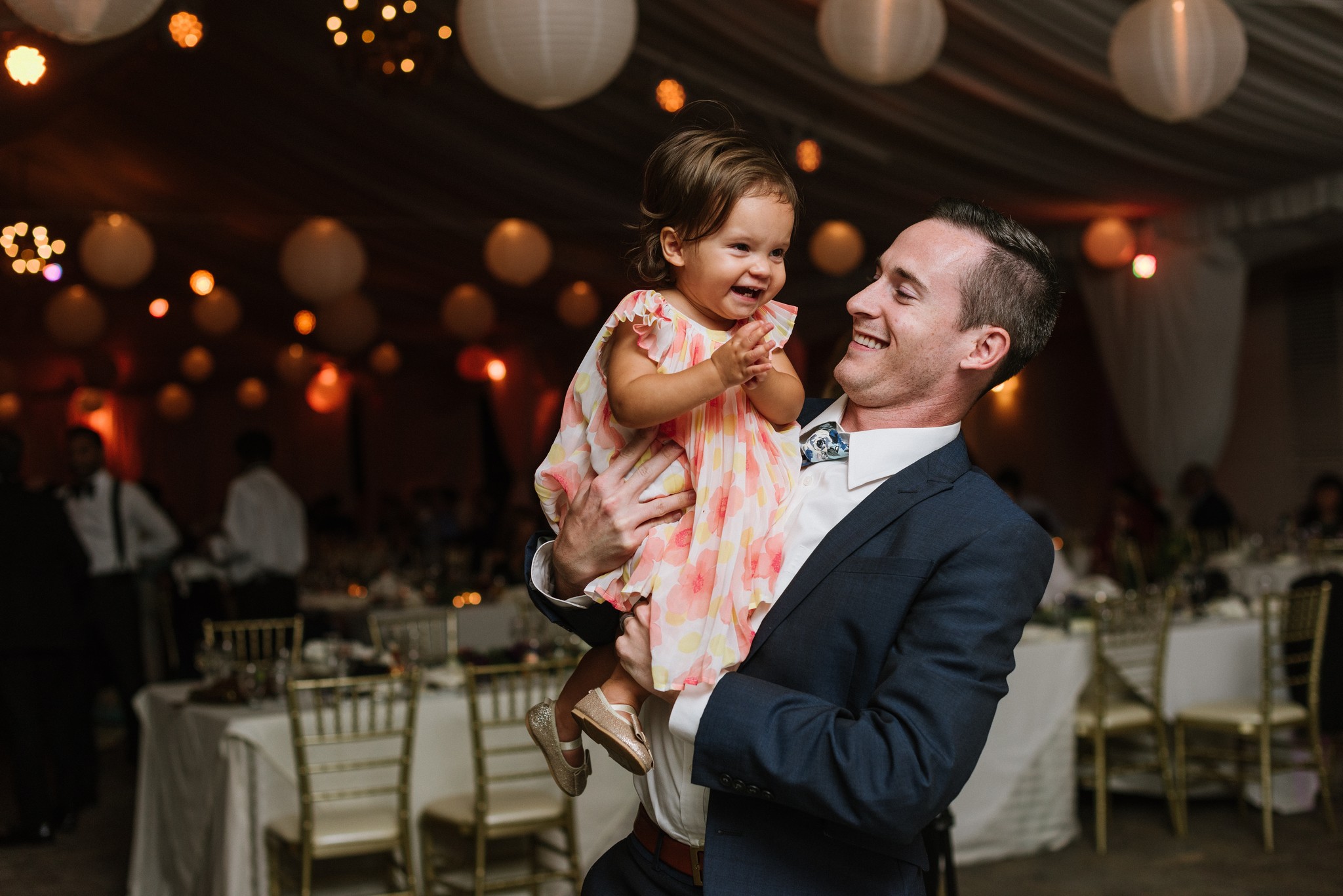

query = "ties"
[[798, 422, 848, 466]]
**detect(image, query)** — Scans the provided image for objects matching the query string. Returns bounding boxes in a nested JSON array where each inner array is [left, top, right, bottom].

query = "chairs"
[[929, 531, 1342, 864], [134, 559, 653, 895]]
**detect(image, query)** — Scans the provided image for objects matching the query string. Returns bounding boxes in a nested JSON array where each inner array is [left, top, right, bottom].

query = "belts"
[[632, 801, 704, 886]]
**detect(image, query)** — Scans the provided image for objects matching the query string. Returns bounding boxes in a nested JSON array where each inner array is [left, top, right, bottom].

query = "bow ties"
[[69, 482, 94, 502]]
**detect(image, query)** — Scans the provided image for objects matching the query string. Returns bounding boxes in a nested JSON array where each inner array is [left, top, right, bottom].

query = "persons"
[[524, 96, 810, 798], [138, 426, 1343, 684], [0, 416, 91, 848], [528, 203, 1069, 896], [46, 424, 181, 812]]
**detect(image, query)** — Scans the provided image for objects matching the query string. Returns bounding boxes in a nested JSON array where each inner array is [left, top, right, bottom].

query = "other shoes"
[[525, 697, 592, 797], [571, 688, 654, 776], [0, 820, 55, 847]]
